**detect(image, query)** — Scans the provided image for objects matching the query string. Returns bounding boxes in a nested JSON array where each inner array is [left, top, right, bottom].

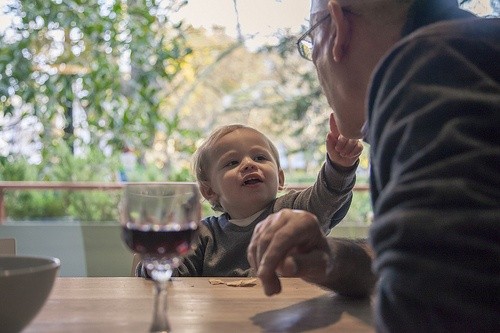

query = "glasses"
[[298, 12, 332, 61]]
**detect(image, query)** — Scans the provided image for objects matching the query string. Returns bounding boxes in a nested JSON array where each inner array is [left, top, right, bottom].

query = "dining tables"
[[21, 276, 379, 333]]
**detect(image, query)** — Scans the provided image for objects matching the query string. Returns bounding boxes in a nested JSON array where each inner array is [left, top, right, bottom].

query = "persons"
[[134, 113, 364, 277], [244, 0, 500, 333]]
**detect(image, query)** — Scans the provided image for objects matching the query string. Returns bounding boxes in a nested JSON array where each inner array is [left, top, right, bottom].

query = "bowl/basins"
[[0, 256, 61, 333]]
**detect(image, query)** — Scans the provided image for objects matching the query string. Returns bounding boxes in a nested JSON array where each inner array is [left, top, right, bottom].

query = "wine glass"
[[118, 182, 203, 333]]
